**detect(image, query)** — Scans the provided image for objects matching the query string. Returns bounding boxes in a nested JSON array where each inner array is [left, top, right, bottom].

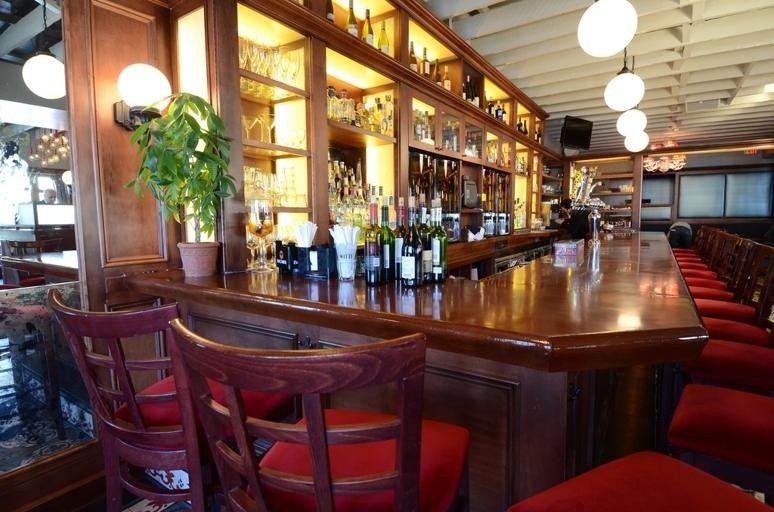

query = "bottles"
[[587, 245, 602, 285], [325, 1, 334, 20], [327, 156, 386, 246], [513, 198, 526, 228], [345, 1, 358, 38], [377, 20, 389, 57], [363, 195, 450, 285], [409, 41, 542, 176], [543, 161, 563, 178], [325, 87, 394, 138], [364, 285, 446, 321], [361, 9, 374, 46], [586, 199, 602, 243]]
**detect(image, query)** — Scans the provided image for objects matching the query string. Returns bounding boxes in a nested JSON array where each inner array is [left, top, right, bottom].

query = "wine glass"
[[239, 37, 301, 99], [247, 198, 278, 272], [241, 112, 305, 150]]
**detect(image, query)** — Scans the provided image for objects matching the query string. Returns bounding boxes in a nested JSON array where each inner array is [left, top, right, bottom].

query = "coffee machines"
[[548, 204, 562, 229]]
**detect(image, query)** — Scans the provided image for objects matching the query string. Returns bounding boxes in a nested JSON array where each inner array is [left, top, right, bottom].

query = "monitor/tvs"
[[463, 180, 478, 208], [560, 115, 593, 150]]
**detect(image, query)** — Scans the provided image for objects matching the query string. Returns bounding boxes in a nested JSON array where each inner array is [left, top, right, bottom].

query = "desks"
[[0, 250, 79, 284], [1, 282, 84, 441]]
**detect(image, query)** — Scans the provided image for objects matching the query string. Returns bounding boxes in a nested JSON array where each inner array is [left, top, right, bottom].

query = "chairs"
[[502, 448, 772, 511], [665, 381, 772, 469], [47, 288, 299, 512], [166, 316, 473, 512], [5, 237, 64, 286], [672, 224, 774, 386]]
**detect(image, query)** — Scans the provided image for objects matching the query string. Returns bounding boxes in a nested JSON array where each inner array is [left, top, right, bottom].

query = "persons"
[[555, 199, 588, 241], [667, 221, 692, 249], [37, 189, 57, 205]]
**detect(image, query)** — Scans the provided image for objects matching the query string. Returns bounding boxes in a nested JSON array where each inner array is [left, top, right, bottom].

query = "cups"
[[243, 164, 307, 209], [338, 284, 356, 308], [620, 185, 633, 193], [532, 216, 543, 230], [542, 184, 555, 194], [337, 254, 355, 283]]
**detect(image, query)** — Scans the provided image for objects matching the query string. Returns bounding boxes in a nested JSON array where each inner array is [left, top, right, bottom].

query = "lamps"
[[114, 63, 173, 131], [22, 0, 67, 100], [28, 128, 70, 169], [643, 155, 687, 174], [577, 0, 649, 152]]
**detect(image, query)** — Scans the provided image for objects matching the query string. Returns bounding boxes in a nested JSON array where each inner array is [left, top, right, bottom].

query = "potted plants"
[[123, 93, 237, 278]]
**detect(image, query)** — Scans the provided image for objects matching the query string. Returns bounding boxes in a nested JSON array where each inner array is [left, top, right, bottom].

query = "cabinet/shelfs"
[[170, 0, 561, 274], [641, 172, 678, 228], [564, 154, 641, 231]]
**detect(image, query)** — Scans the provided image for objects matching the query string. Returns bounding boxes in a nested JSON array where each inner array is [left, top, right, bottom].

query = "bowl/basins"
[[608, 187, 619, 192]]
